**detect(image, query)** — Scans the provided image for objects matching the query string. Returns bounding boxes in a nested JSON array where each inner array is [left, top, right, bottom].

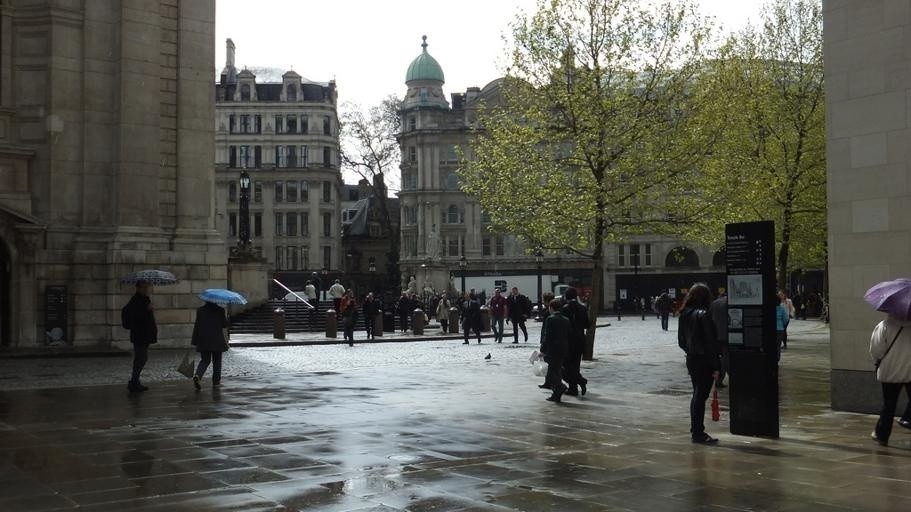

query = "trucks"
[[450, 275, 588, 318]]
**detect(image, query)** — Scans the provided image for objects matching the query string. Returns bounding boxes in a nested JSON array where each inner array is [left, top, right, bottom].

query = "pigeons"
[[484, 353, 491, 360]]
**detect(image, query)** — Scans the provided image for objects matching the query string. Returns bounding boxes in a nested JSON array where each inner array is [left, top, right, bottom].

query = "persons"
[[339, 288, 359, 346], [774, 289, 810, 361], [870, 317, 911, 445], [361, 287, 476, 339], [484, 291, 533, 327], [710, 292, 730, 388], [459, 292, 482, 344], [328, 279, 346, 320], [305, 278, 317, 321], [537, 287, 590, 402], [677, 281, 721, 445], [490, 288, 508, 344], [508, 287, 528, 344], [127, 279, 161, 393], [631, 288, 683, 331], [311, 270, 321, 302], [191, 302, 228, 389]]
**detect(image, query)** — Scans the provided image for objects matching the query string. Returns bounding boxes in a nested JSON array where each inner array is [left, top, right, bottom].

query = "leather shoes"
[[896, 417, 911, 429], [539, 380, 554, 390], [564, 386, 579, 396], [579, 377, 589, 395], [871, 430, 890, 445], [546, 385, 567, 401]]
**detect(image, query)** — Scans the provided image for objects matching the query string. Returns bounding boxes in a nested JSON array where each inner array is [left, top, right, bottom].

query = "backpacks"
[[121, 295, 140, 330]]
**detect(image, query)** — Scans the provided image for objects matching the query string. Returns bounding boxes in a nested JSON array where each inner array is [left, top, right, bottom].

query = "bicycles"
[[818, 292, 829, 323]]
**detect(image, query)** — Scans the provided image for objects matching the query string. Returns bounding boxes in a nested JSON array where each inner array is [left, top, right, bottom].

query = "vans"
[[792, 292, 821, 314]]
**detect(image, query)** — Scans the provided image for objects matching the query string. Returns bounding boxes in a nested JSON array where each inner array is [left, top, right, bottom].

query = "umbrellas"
[[862, 275, 911, 322], [196, 288, 248, 306], [120, 269, 181, 288]]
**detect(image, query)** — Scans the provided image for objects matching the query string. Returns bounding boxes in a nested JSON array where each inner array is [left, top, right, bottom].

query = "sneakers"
[[128, 380, 149, 393], [193, 375, 202, 390], [213, 382, 225, 388], [692, 433, 720, 444]]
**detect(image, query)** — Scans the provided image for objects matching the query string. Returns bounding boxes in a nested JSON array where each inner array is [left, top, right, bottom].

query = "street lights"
[[322, 263, 328, 300], [535, 247, 544, 321], [239, 169, 251, 263], [459, 255, 469, 296]]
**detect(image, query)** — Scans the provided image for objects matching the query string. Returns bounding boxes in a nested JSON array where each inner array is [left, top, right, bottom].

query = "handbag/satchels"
[[873, 359, 883, 378], [533, 360, 550, 378], [177, 356, 196, 379]]
